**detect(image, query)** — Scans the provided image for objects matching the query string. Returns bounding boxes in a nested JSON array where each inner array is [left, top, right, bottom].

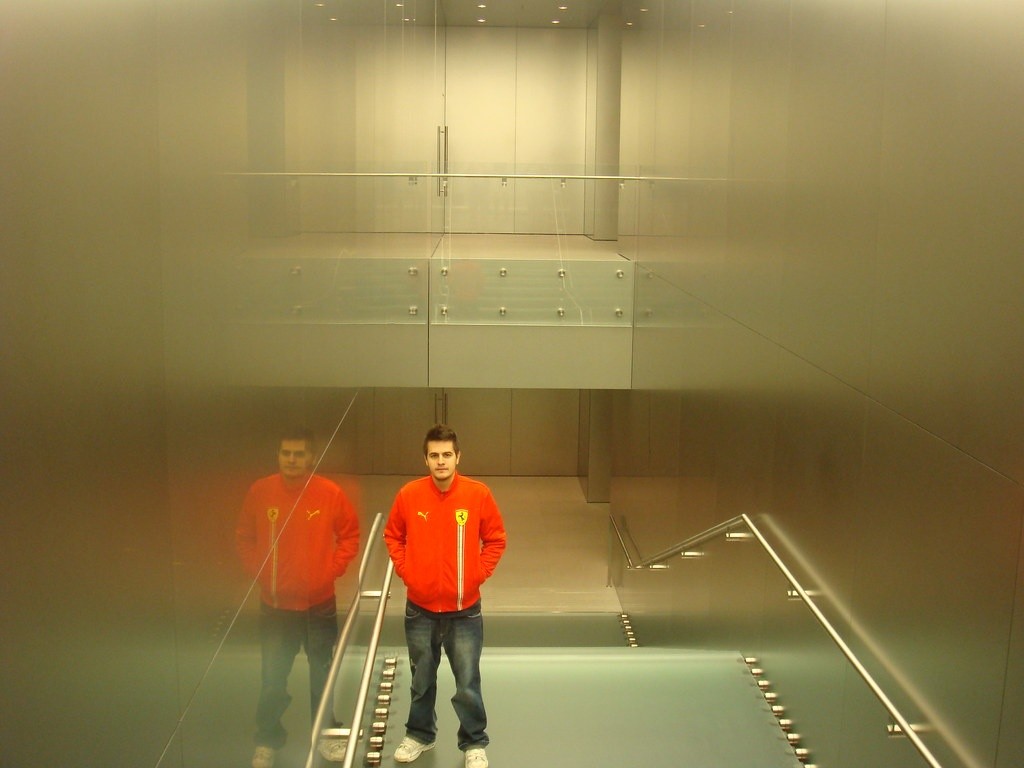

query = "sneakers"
[[464, 749, 489, 768], [317, 737, 347, 762], [393, 735, 437, 763], [251, 746, 275, 768]]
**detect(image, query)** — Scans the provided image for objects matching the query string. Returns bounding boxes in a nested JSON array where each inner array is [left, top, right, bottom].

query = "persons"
[[382, 423, 509, 768], [237, 427, 359, 768]]
